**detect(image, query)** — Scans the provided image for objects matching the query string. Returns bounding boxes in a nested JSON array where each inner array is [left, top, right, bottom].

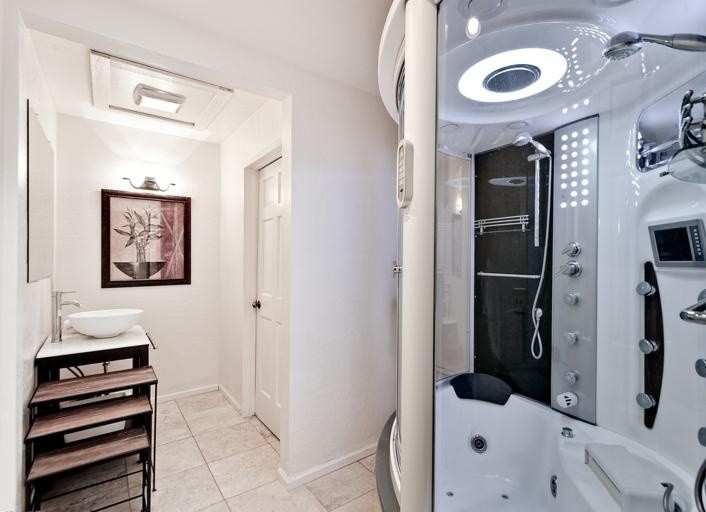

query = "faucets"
[[51, 291, 80, 343]]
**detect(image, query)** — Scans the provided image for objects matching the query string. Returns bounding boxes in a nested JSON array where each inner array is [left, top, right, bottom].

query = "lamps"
[[133, 83, 185, 115]]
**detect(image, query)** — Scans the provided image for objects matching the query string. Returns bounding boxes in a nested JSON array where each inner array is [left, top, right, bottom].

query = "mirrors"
[[26, 99, 54, 283]]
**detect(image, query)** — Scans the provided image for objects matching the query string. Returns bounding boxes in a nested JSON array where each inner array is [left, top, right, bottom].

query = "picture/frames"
[[100, 189, 191, 288]]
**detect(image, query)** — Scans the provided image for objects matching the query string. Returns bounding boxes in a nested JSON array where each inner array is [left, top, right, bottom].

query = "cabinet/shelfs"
[[25, 366, 158, 511]]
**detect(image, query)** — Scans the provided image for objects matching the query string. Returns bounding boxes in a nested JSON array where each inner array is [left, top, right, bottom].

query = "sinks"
[[67, 309, 143, 339]]
